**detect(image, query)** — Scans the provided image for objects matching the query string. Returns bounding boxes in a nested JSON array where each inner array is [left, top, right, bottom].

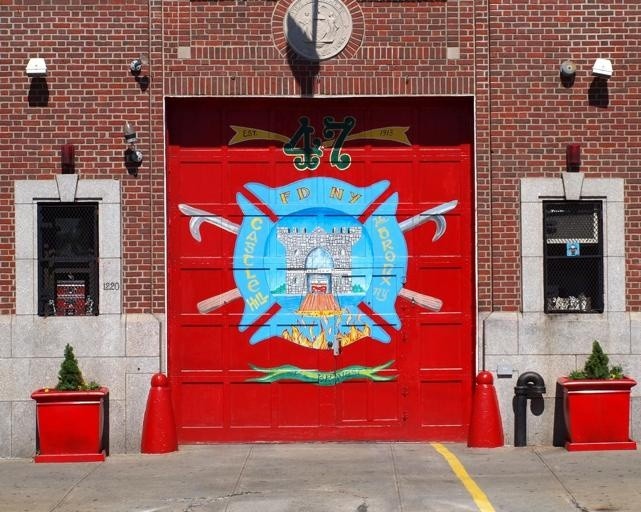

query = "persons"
[[325, 11, 339, 40], [298, 11, 312, 41]]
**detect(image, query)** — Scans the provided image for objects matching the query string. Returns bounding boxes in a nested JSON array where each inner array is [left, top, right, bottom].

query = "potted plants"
[[30, 343, 110, 464], [557, 339, 637, 451]]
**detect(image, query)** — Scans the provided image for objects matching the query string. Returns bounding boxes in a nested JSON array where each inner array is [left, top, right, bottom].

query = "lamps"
[[589, 57, 614, 80], [24, 56, 47, 76]]
